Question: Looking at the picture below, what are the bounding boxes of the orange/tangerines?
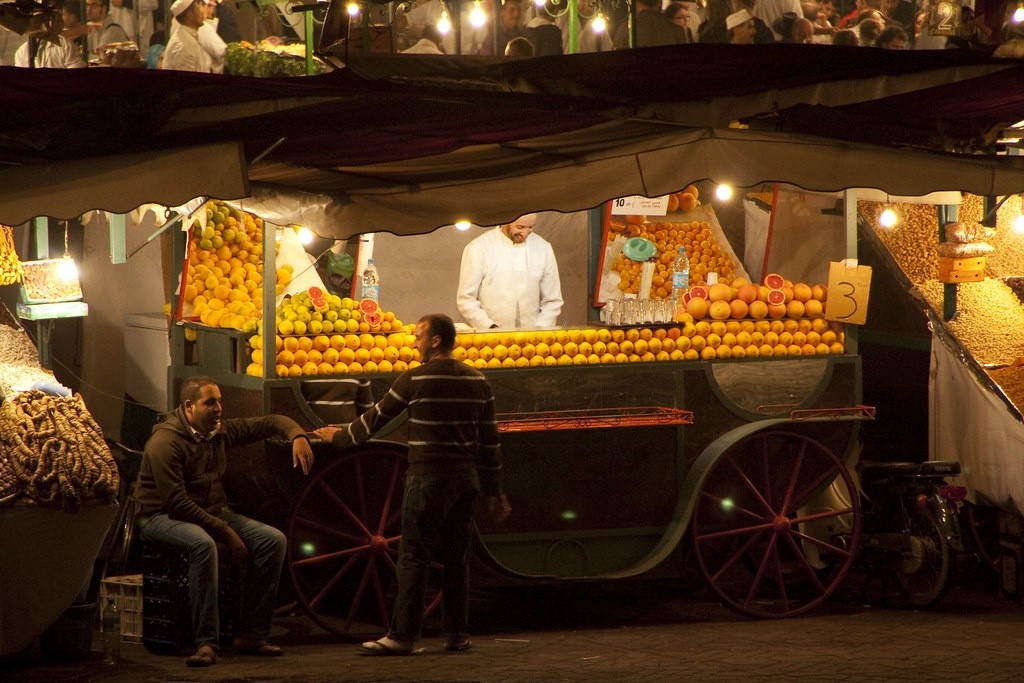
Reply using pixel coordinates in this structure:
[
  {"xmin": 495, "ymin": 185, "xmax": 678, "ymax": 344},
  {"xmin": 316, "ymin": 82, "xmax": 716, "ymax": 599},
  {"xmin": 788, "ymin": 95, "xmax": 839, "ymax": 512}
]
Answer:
[
  {"xmin": 608, "ymin": 184, "xmax": 738, "ymax": 298},
  {"xmin": 246, "ymin": 334, "xmax": 423, "ymax": 378},
  {"xmin": 448, "ymin": 319, "xmax": 846, "ymax": 369},
  {"xmin": 675, "ymin": 274, "xmax": 829, "ymax": 323},
  {"xmin": 182, "ymin": 201, "xmax": 416, "ymax": 333}
]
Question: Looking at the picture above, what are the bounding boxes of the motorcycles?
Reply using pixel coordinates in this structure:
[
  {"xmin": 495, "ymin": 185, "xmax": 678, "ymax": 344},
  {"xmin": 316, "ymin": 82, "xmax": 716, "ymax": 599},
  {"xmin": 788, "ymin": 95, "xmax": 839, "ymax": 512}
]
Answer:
[{"xmin": 737, "ymin": 461, "xmax": 967, "ymax": 613}]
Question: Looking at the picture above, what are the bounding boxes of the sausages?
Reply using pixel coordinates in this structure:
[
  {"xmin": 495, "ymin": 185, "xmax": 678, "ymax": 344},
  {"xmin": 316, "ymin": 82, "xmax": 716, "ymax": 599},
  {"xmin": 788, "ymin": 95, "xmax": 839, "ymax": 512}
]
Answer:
[{"xmin": 0, "ymin": 389, "xmax": 120, "ymax": 520}]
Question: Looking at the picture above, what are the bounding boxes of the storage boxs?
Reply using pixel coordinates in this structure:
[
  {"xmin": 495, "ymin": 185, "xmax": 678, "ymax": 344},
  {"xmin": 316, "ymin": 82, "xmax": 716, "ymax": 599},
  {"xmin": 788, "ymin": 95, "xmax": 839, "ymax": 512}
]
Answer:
[
  {"xmin": 97, "ymin": 571, "xmax": 142, "ymax": 646},
  {"xmin": 14, "ymin": 258, "xmax": 88, "ymax": 319},
  {"xmin": 939, "ymin": 257, "xmax": 986, "ymax": 284},
  {"xmin": 143, "ymin": 540, "xmax": 238, "ymax": 654}
]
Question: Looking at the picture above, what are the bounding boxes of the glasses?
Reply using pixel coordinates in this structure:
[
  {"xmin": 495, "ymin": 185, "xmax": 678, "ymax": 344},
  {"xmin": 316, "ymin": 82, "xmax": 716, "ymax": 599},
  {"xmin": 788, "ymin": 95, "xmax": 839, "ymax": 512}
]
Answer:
[{"xmin": 672, "ymin": 15, "xmax": 690, "ymax": 20}]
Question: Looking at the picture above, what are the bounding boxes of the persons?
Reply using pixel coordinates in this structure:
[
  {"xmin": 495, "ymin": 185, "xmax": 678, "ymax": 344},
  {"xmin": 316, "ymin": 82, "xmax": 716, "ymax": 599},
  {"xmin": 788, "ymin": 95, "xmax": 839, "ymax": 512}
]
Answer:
[
  {"xmin": 0, "ymin": 0, "xmax": 154, "ymax": 67},
  {"xmin": 395, "ymin": 0, "xmax": 774, "ymax": 56},
  {"xmin": 135, "ymin": 376, "xmax": 315, "ymax": 663},
  {"xmin": 753, "ymin": 0, "xmax": 1024, "ymax": 51},
  {"xmin": 457, "ymin": 212, "xmax": 564, "ymax": 329},
  {"xmin": 162, "ymin": 0, "xmax": 236, "ymax": 74},
  {"xmin": 314, "ymin": 317, "xmax": 511, "ymax": 654}
]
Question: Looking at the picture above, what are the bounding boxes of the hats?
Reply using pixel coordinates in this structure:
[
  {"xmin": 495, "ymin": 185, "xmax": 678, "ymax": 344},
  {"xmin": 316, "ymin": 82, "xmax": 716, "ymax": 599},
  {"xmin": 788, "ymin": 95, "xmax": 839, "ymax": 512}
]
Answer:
[
  {"xmin": 726, "ymin": 8, "xmax": 751, "ymax": 31},
  {"xmin": 170, "ymin": 0, "xmax": 194, "ymax": 16}
]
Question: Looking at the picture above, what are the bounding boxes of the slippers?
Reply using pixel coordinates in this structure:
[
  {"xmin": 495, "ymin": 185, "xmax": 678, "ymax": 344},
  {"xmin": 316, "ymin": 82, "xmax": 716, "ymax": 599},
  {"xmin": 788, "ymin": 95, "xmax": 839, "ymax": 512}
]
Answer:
[
  {"xmin": 233, "ymin": 640, "xmax": 284, "ymax": 656},
  {"xmin": 357, "ymin": 640, "xmax": 411, "ymax": 655},
  {"xmin": 185, "ymin": 650, "xmax": 217, "ymax": 667},
  {"xmin": 445, "ymin": 639, "xmax": 472, "ymax": 651}
]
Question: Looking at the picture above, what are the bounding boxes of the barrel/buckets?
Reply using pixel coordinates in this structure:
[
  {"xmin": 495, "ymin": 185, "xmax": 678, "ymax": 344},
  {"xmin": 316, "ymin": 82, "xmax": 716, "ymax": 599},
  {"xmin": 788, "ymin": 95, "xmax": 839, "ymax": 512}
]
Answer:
[{"xmin": 41, "ymin": 600, "xmax": 98, "ymax": 666}]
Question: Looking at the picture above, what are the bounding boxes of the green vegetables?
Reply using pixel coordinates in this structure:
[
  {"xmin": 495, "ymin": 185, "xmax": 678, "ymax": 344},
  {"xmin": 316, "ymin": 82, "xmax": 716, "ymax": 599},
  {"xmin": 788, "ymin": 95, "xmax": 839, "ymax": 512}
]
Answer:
[{"xmin": 224, "ymin": 42, "xmax": 325, "ymax": 76}]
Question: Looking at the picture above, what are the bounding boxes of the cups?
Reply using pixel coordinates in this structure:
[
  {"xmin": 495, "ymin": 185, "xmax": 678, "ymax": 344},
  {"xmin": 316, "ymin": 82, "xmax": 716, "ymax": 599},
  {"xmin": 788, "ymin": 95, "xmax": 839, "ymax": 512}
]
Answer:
[{"xmin": 606, "ymin": 298, "xmax": 678, "ymax": 325}]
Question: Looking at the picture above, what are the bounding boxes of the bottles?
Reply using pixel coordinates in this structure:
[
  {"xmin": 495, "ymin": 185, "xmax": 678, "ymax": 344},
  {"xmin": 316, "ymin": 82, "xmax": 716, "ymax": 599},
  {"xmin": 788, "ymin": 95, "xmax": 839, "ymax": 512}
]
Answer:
[
  {"xmin": 362, "ymin": 259, "xmax": 379, "ymax": 305},
  {"xmin": 102, "ymin": 597, "xmax": 121, "ymax": 665},
  {"xmin": 672, "ymin": 246, "xmax": 690, "ymax": 298},
  {"xmin": 934, "ymin": 490, "xmax": 961, "ymax": 551}
]
